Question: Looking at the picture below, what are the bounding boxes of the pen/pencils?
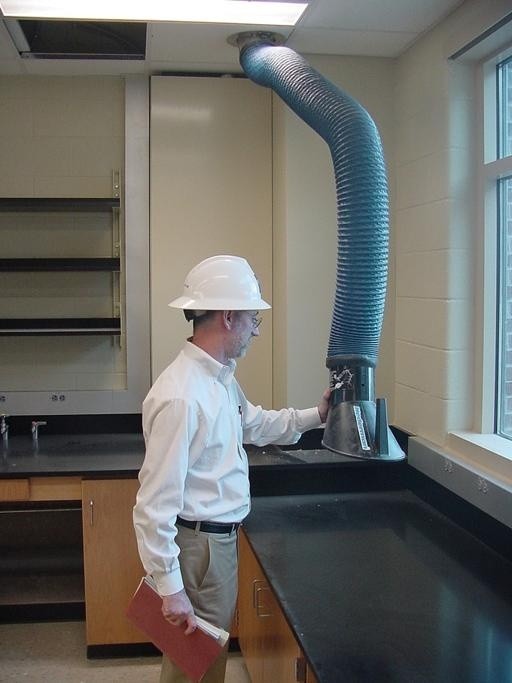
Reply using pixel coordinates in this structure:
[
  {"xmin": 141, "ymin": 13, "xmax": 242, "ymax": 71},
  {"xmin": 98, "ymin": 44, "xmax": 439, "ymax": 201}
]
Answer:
[{"xmin": 238, "ymin": 405, "xmax": 243, "ymax": 428}]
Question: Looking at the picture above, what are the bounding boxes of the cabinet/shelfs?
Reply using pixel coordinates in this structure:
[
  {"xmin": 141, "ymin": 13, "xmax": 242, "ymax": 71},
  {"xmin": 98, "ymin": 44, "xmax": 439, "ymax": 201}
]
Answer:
[
  {"xmin": 81, "ymin": 480, "xmax": 155, "ymax": 643},
  {"xmin": 0, "ymin": 74, "xmax": 125, "ymax": 335},
  {"xmin": 233, "ymin": 526, "xmax": 298, "ymax": 683}
]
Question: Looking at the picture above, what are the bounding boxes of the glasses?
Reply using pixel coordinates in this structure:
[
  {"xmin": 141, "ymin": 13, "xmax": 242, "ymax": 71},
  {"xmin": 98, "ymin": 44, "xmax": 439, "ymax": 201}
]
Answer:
[{"xmin": 242, "ymin": 311, "xmax": 263, "ymax": 328}]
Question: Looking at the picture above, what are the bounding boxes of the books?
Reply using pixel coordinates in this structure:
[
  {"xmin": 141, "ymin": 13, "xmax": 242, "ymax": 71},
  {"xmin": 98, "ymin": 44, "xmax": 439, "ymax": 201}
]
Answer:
[{"xmin": 122, "ymin": 572, "xmax": 230, "ymax": 683}]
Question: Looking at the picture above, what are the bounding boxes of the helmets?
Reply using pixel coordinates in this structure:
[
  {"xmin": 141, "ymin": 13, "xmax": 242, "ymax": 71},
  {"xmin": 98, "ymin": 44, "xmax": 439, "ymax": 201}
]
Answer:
[{"xmin": 167, "ymin": 253, "xmax": 274, "ymax": 314}]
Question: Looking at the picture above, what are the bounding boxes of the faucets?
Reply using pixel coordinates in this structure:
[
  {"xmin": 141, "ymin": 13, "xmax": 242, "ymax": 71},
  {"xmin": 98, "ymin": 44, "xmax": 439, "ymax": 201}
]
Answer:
[{"xmin": 0, "ymin": 415, "xmax": 9, "ymax": 441}]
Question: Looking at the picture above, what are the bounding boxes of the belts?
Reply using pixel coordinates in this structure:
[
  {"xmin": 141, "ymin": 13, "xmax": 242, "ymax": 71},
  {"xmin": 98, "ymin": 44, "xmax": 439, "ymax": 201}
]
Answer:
[{"xmin": 175, "ymin": 513, "xmax": 241, "ymax": 535}]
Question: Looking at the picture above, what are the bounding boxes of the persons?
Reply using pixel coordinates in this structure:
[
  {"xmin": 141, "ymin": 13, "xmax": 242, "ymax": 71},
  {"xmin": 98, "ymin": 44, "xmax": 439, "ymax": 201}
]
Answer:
[{"xmin": 131, "ymin": 252, "xmax": 334, "ymax": 683}]
